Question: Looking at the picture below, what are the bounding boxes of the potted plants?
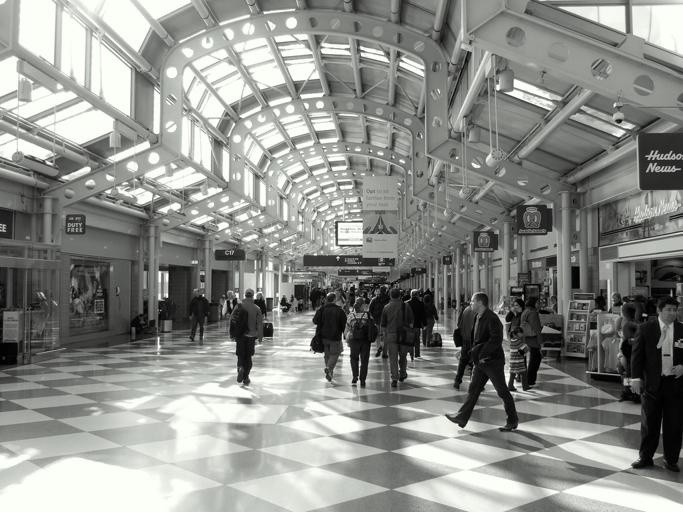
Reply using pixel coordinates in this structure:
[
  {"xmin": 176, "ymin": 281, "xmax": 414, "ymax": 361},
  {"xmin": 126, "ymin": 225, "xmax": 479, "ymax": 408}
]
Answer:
[{"xmin": 160, "ymin": 297, "xmax": 180, "ymax": 332}]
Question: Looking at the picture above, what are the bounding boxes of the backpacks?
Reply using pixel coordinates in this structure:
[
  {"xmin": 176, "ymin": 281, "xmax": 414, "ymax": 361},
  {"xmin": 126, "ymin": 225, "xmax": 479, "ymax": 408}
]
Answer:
[{"xmin": 353, "ymin": 312, "xmax": 366, "ymax": 340}]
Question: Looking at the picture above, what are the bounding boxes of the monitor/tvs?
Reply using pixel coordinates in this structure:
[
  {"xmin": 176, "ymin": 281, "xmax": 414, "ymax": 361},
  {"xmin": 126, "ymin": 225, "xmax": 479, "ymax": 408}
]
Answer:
[{"xmin": 335, "ymin": 220, "xmax": 364, "ymax": 246}]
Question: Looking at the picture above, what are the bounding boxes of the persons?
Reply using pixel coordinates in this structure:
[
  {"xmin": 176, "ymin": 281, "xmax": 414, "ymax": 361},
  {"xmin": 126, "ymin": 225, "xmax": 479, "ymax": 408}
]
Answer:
[
  {"xmin": 309, "ymin": 283, "xmax": 439, "ymax": 387},
  {"xmin": 229, "ymin": 288, "xmax": 264, "ymax": 385},
  {"xmin": 220, "ymin": 290, "xmax": 241, "ymax": 339},
  {"xmin": 590, "ymin": 296, "xmax": 606, "ymax": 329},
  {"xmin": 609, "ymin": 293, "xmax": 623, "ymax": 313},
  {"xmin": 254, "ymin": 292, "xmax": 267, "ymax": 320},
  {"xmin": 550, "ymin": 296, "xmax": 558, "ymax": 314},
  {"xmin": 444, "ymin": 292, "xmax": 519, "ymax": 431},
  {"xmin": 281, "ymin": 294, "xmax": 304, "ymax": 312},
  {"xmin": 632, "ymin": 296, "xmax": 683, "ymax": 473},
  {"xmin": 189, "ymin": 288, "xmax": 210, "ymax": 341},
  {"xmin": 506, "ymin": 296, "xmax": 543, "ymax": 390}
]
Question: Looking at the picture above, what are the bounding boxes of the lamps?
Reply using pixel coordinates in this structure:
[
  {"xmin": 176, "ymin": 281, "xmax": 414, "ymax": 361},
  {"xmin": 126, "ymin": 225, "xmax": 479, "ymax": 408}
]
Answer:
[
  {"xmin": 112, "ymin": 187, "xmax": 137, "ymax": 203},
  {"xmin": 17, "ymin": 78, "xmax": 31, "ymax": 101},
  {"xmin": 109, "ymin": 132, "xmax": 122, "ymax": 149},
  {"xmin": 14, "ymin": 150, "xmax": 60, "ymax": 178},
  {"xmin": 164, "ymin": 162, "xmax": 259, "ymax": 245},
  {"xmin": 399, "ymin": 65, "xmax": 515, "ymax": 268}
]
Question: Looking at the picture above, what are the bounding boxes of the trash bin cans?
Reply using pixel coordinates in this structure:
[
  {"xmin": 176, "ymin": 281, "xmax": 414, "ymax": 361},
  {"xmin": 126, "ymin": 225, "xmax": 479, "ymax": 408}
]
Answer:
[{"xmin": 208, "ymin": 303, "xmax": 221, "ymax": 321}]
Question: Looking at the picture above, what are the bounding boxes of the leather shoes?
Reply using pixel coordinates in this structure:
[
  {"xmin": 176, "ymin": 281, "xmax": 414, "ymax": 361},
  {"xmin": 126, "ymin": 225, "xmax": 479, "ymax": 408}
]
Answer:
[
  {"xmin": 445, "ymin": 414, "xmax": 466, "ymax": 428},
  {"xmin": 498, "ymin": 421, "xmax": 517, "ymax": 431},
  {"xmin": 663, "ymin": 458, "xmax": 680, "ymax": 473},
  {"xmin": 631, "ymin": 458, "xmax": 653, "ymax": 467}
]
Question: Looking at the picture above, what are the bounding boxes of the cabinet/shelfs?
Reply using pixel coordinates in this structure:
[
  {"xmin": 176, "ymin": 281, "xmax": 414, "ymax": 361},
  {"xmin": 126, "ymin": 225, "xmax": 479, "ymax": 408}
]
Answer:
[{"xmin": 564, "ymin": 301, "xmax": 595, "ymax": 358}]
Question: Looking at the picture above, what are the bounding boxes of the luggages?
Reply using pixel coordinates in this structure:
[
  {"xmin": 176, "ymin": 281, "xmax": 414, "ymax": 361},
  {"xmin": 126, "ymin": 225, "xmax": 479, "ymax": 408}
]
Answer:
[
  {"xmin": 263, "ymin": 319, "xmax": 273, "ymax": 337},
  {"xmin": 430, "ymin": 319, "xmax": 442, "ymax": 347}
]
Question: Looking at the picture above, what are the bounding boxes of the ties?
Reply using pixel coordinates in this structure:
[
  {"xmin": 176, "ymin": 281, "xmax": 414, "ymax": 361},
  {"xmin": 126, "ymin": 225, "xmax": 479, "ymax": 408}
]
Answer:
[{"xmin": 657, "ymin": 327, "xmax": 672, "ymax": 377}]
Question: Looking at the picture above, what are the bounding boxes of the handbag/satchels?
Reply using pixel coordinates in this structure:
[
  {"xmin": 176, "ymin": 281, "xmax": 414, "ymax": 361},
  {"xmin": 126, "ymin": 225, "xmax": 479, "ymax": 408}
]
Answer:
[
  {"xmin": 453, "ymin": 328, "xmax": 462, "ymax": 347},
  {"xmin": 402, "ymin": 329, "xmax": 416, "ymax": 346},
  {"xmin": 367, "ymin": 319, "xmax": 378, "ymax": 342},
  {"xmin": 311, "ymin": 325, "xmax": 324, "ymax": 352},
  {"xmin": 231, "ymin": 307, "xmax": 248, "ymax": 337}
]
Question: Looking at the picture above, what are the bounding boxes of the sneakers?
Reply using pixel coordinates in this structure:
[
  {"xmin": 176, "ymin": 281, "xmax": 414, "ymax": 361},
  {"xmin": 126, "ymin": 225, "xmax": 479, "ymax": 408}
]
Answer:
[
  {"xmin": 509, "ymin": 386, "xmax": 533, "ymax": 391},
  {"xmin": 352, "ymin": 376, "xmax": 358, "ymax": 383},
  {"xmin": 376, "ymin": 348, "xmax": 382, "ymax": 357},
  {"xmin": 383, "ymin": 354, "xmax": 388, "ymax": 358},
  {"xmin": 325, "ymin": 367, "xmax": 331, "ymax": 382},
  {"xmin": 400, "ymin": 373, "xmax": 407, "ymax": 382},
  {"xmin": 237, "ymin": 366, "xmax": 250, "ymax": 383},
  {"xmin": 190, "ymin": 335, "xmax": 193, "ymax": 340},
  {"xmin": 453, "ymin": 383, "xmax": 460, "ymax": 390},
  {"xmin": 391, "ymin": 380, "xmax": 397, "ymax": 386},
  {"xmin": 361, "ymin": 378, "xmax": 365, "ymax": 385}
]
